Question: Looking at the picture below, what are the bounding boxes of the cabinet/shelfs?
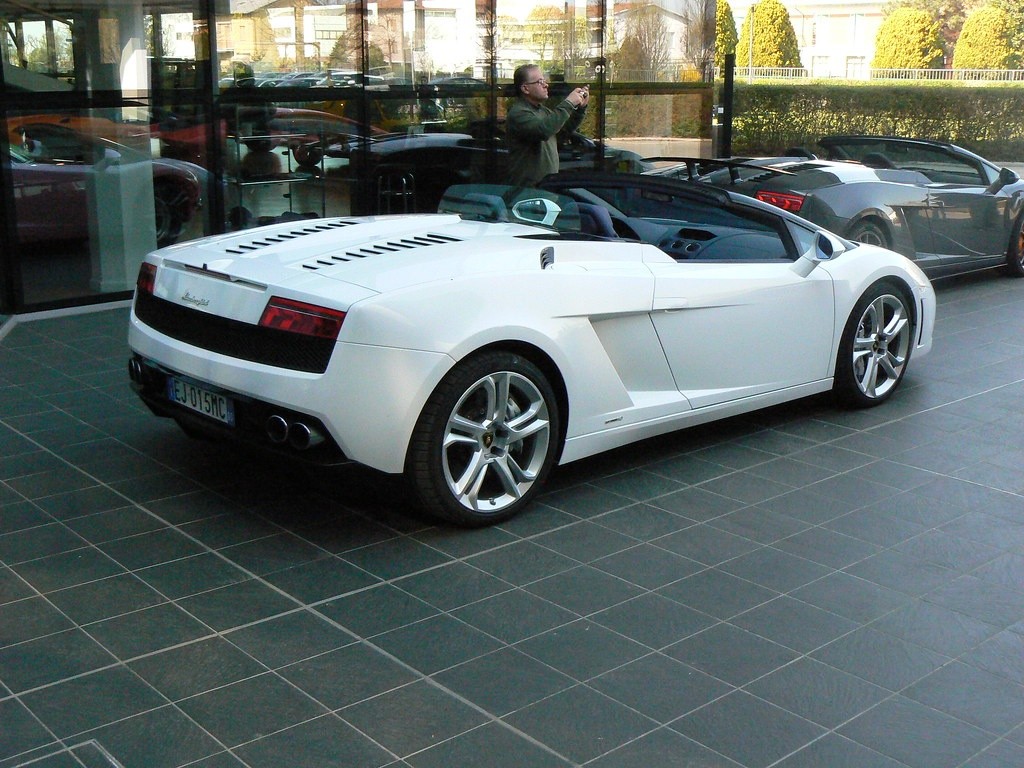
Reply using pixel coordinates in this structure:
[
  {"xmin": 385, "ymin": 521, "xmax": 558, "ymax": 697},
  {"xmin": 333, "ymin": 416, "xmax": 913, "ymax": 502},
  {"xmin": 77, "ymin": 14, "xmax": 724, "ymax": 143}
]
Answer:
[{"xmin": 225, "ymin": 38, "xmax": 326, "ymax": 223}]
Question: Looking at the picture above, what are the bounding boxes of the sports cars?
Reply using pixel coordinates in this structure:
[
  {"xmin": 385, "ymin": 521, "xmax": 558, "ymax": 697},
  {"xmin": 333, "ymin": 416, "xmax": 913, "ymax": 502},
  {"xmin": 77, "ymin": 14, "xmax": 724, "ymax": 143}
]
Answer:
[
  {"xmin": 1, "ymin": 71, "xmax": 656, "ymax": 251},
  {"xmin": 126, "ymin": 167, "xmax": 938, "ymax": 529},
  {"xmin": 638, "ymin": 136, "xmax": 1024, "ymax": 282}
]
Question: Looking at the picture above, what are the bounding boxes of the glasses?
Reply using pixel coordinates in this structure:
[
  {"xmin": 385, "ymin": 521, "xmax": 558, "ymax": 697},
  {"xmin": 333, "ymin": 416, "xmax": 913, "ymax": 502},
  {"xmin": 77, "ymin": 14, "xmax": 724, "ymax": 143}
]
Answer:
[{"xmin": 525, "ymin": 78, "xmax": 547, "ymax": 85}]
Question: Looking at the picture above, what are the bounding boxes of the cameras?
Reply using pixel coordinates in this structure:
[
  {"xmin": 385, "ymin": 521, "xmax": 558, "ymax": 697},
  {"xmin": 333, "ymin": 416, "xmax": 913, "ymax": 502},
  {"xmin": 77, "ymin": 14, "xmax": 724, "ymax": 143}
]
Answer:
[{"xmin": 580, "ymin": 91, "xmax": 588, "ymax": 99}]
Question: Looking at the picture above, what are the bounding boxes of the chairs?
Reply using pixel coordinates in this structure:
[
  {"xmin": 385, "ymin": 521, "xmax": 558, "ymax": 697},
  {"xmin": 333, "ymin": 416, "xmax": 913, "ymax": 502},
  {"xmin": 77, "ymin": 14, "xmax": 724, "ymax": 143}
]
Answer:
[
  {"xmin": 462, "ymin": 194, "xmax": 508, "ymax": 222},
  {"xmin": 553, "ymin": 202, "xmax": 620, "ymax": 238},
  {"xmin": 785, "ymin": 146, "xmax": 817, "ymax": 160},
  {"xmin": 861, "ymin": 153, "xmax": 897, "ymax": 170}
]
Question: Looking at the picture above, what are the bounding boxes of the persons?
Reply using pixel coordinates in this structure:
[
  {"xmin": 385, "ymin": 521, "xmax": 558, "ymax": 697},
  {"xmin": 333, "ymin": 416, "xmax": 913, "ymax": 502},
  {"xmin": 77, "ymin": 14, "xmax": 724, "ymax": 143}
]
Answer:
[
  {"xmin": 218, "ymin": 62, "xmax": 277, "ymax": 154},
  {"xmin": 417, "ymin": 76, "xmax": 440, "ymax": 120},
  {"xmin": 505, "ymin": 63, "xmax": 589, "ymax": 187}
]
